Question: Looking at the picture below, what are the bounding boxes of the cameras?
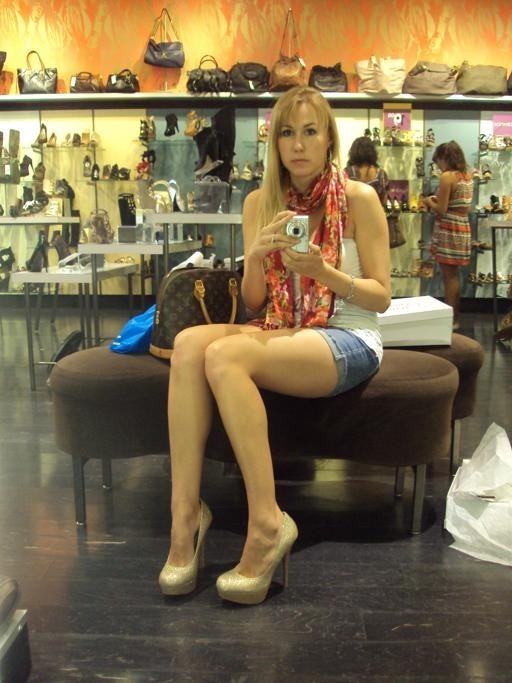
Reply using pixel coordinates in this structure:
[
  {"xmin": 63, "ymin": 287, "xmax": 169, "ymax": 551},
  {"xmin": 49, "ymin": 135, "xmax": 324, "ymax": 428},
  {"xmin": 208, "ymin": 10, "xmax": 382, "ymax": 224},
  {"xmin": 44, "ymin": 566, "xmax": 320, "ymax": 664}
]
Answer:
[{"xmin": 286, "ymin": 214, "xmax": 309, "ymax": 254}]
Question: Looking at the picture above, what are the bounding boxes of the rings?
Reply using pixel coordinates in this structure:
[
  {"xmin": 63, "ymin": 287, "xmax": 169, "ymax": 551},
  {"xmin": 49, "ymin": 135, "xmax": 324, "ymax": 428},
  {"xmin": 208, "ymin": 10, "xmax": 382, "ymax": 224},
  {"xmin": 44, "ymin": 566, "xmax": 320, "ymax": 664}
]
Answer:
[{"xmin": 271, "ymin": 232, "xmax": 274, "ymax": 241}]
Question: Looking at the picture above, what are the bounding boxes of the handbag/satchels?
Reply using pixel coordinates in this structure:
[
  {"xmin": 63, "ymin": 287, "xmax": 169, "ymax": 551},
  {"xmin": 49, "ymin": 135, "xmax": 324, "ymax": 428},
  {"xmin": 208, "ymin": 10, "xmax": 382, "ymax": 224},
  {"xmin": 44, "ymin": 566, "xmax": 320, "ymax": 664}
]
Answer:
[
  {"xmin": 226, "ymin": 62, "xmax": 269, "ymax": 93},
  {"xmin": 149, "ymin": 267, "xmax": 245, "ymax": 359},
  {"xmin": 268, "ymin": 8, "xmax": 306, "ymax": 93},
  {"xmin": 70, "ymin": 69, "xmax": 139, "ymax": 93},
  {"xmin": 144, "ymin": 8, "xmax": 184, "ymax": 67},
  {"xmin": 456, "ymin": 61, "xmax": 508, "ymax": 95},
  {"xmin": 187, "ymin": 55, "xmax": 228, "ymax": 93},
  {"xmin": 401, "ymin": 61, "xmax": 459, "ymax": 95},
  {"xmin": 386, "ymin": 210, "xmax": 405, "ymax": 248},
  {"xmin": 17, "ymin": 51, "xmax": 56, "ymax": 94},
  {"xmin": 357, "ymin": 55, "xmax": 406, "ymax": 94},
  {"xmin": 309, "ymin": 63, "xmax": 348, "ymax": 92}
]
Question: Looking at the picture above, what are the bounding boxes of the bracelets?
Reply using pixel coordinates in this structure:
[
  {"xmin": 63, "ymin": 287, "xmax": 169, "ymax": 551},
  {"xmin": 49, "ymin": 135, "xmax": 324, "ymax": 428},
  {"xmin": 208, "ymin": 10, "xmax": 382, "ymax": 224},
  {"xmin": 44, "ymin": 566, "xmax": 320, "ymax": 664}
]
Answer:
[{"xmin": 337, "ymin": 275, "xmax": 358, "ymax": 303}]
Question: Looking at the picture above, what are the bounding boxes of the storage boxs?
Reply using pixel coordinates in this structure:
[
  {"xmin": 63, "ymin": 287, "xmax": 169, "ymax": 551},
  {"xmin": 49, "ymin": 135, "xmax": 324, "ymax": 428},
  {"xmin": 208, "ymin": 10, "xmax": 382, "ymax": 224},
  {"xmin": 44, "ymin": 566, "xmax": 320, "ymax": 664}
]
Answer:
[{"xmin": 377, "ymin": 294, "xmax": 455, "ymax": 348}]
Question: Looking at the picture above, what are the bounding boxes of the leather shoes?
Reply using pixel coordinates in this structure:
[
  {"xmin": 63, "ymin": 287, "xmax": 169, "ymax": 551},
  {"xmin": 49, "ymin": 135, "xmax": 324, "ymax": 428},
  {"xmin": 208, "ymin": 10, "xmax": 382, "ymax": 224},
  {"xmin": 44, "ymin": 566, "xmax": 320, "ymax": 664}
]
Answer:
[
  {"xmin": 83, "ymin": 155, "xmax": 130, "ymax": 181},
  {"xmin": 38, "ymin": 124, "xmax": 90, "ymax": 147},
  {"xmin": 231, "ymin": 160, "xmax": 263, "ymax": 181},
  {"xmin": 8, "ymin": 183, "xmax": 48, "ymax": 216}
]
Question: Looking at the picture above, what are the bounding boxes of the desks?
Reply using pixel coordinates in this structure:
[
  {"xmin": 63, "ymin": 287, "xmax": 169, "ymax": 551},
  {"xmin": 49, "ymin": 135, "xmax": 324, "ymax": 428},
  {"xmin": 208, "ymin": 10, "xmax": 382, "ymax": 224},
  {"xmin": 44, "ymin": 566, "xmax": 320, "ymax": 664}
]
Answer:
[
  {"xmin": 490, "ymin": 222, "xmax": 512, "ymax": 332},
  {"xmin": 10, "ymin": 214, "xmax": 79, "ymax": 331},
  {"xmin": 78, "ymin": 240, "xmax": 203, "ymax": 352},
  {"xmin": 11, "ymin": 261, "xmax": 139, "ymax": 392},
  {"xmin": 145, "ymin": 211, "xmax": 247, "ymax": 276}
]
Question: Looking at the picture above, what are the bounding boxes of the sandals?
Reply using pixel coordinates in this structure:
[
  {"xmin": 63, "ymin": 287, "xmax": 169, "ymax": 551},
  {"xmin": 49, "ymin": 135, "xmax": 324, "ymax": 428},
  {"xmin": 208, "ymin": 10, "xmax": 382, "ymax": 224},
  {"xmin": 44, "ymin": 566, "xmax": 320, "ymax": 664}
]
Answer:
[
  {"xmin": 472, "ymin": 194, "xmax": 512, "ymax": 218},
  {"xmin": 386, "ymin": 195, "xmax": 442, "ymax": 213},
  {"xmin": 391, "ymin": 263, "xmax": 512, "ymax": 284},
  {"xmin": 470, "ymin": 240, "xmax": 492, "ymax": 254},
  {"xmin": 479, "ymin": 133, "xmax": 512, "ymax": 151},
  {"xmin": 416, "ymin": 157, "xmax": 492, "ymax": 178},
  {"xmin": 365, "ymin": 126, "xmax": 434, "ymax": 146}
]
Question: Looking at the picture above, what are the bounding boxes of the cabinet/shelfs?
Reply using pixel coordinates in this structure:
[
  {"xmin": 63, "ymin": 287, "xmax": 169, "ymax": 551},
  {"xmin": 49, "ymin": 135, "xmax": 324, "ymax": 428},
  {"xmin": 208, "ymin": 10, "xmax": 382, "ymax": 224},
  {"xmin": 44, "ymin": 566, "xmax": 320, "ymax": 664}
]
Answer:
[{"xmin": 0, "ymin": 87, "xmax": 512, "ymax": 286}]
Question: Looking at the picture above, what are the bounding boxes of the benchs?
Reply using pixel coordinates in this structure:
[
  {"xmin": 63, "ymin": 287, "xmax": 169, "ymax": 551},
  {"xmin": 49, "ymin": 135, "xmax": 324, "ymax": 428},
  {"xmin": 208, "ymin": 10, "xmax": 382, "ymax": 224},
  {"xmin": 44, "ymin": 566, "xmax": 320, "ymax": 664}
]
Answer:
[
  {"xmin": 46, "ymin": 350, "xmax": 460, "ymax": 536},
  {"xmin": 224, "ymin": 329, "xmax": 484, "ymax": 468}
]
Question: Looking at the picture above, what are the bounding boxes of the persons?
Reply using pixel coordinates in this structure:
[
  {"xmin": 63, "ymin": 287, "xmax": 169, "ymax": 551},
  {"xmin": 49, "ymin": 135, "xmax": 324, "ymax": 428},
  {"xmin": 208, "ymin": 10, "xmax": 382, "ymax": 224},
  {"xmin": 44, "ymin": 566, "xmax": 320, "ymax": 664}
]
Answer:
[
  {"xmin": 156, "ymin": 82, "xmax": 392, "ymax": 606},
  {"xmin": 342, "ymin": 133, "xmax": 390, "ymax": 216},
  {"xmin": 419, "ymin": 139, "xmax": 475, "ymax": 330}
]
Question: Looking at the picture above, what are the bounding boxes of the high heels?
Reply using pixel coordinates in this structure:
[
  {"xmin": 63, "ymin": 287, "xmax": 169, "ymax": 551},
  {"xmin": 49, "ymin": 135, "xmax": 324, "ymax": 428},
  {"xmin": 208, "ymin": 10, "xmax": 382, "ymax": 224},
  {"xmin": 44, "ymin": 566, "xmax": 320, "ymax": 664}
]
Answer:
[
  {"xmin": 20, "ymin": 155, "xmax": 45, "ymax": 180},
  {"xmin": 158, "ymin": 498, "xmax": 212, "ymax": 595},
  {"xmin": 26, "ymin": 235, "xmax": 74, "ymax": 272},
  {"xmin": 194, "ymin": 131, "xmax": 217, "ymax": 173},
  {"xmin": 150, "ymin": 180, "xmax": 185, "ymax": 213},
  {"xmin": 216, "ymin": 511, "xmax": 298, "ymax": 604},
  {"xmin": 140, "ymin": 111, "xmax": 201, "ymax": 141}
]
